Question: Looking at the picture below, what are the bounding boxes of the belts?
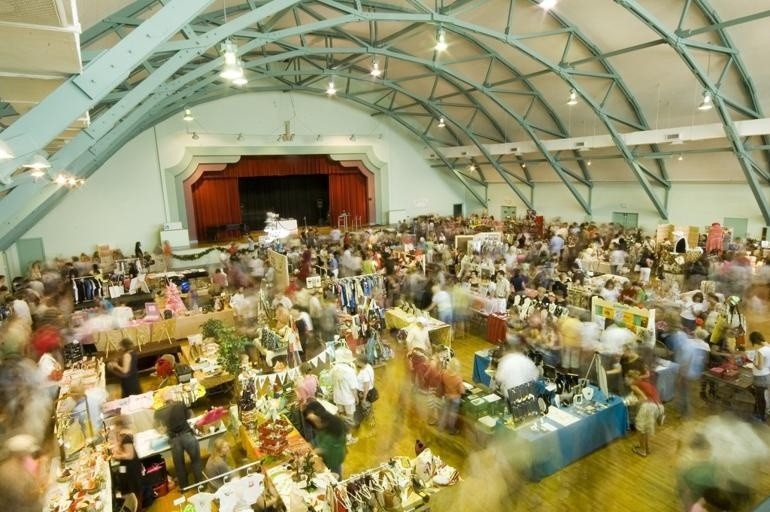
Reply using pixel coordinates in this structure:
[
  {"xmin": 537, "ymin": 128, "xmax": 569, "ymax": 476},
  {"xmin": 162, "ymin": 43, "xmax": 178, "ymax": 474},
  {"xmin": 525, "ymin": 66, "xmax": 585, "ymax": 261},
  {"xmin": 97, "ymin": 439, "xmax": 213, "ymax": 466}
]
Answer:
[{"xmin": 171, "ymin": 428, "xmax": 191, "ymax": 439}]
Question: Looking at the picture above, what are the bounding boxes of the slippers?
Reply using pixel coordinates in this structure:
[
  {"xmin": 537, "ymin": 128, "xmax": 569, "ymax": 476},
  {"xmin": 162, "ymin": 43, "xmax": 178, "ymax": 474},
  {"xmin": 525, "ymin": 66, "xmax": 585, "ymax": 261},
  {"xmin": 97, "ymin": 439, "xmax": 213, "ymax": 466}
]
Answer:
[{"xmin": 632, "ymin": 444, "xmax": 652, "ymax": 457}]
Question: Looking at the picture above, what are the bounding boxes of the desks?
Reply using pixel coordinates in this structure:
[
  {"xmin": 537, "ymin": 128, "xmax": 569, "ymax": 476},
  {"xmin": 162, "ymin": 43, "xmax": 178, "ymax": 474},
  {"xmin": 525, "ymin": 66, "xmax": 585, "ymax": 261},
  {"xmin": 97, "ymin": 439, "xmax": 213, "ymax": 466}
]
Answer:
[
  {"xmin": 460, "ymin": 286, "xmax": 681, "ymax": 478},
  {"xmin": 42, "ymin": 285, "xmax": 465, "ymax": 511},
  {"xmin": 700, "ymin": 353, "xmax": 753, "ymax": 405}
]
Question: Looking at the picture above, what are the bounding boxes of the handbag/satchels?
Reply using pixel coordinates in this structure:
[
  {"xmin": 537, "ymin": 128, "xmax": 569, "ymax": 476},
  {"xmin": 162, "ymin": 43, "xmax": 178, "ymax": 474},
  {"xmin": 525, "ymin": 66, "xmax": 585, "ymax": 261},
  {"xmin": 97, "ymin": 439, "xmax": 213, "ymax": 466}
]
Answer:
[
  {"xmin": 332, "ymin": 469, "xmax": 402, "ymax": 512},
  {"xmin": 367, "ymin": 389, "xmax": 379, "ymax": 402},
  {"xmin": 351, "ymin": 299, "xmax": 386, "ymax": 340},
  {"xmin": 657, "ymin": 414, "xmax": 666, "ymax": 426},
  {"xmin": 710, "ymin": 313, "xmax": 746, "ymax": 352}
]
